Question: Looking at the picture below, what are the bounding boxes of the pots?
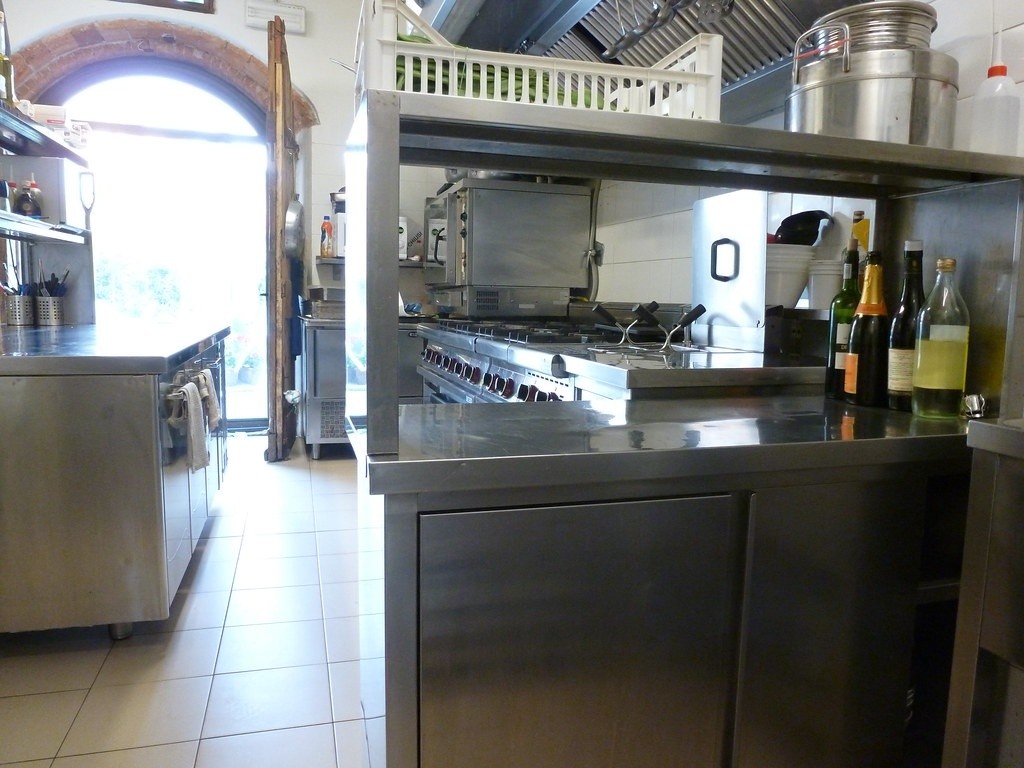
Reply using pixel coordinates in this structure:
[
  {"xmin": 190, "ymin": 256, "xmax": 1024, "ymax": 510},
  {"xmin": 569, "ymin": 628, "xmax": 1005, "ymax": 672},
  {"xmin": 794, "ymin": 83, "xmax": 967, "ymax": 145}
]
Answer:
[{"xmin": 783, "ymin": 21, "xmax": 959, "ymax": 150}]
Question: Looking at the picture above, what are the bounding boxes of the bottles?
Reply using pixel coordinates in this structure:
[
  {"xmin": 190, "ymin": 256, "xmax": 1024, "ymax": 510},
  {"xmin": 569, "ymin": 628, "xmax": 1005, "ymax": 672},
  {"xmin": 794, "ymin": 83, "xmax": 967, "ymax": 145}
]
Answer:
[
  {"xmin": 970, "ymin": 23, "xmax": 1020, "ymax": 157},
  {"xmin": 0, "ymin": 52, "xmax": 12, "ymax": 106},
  {"xmin": 320, "ymin": 215, "xmax": 334, "ymax": 257},
  {"xmin": 28, "ymin": 173, "xmax": 43, "ymax": 213},
  {"xmin": 887, "ymin": 240, "xmax": 934, "ymax": 410},
  {"xmin": 840, "ymin": 211, "xmax": 870, "ymax": 294},
  {"xmin": 843, "ymin": 251, "xmax": 892, "ymax": 407},
  {"xmin": 824, "ymin": 251, "xmax": 864, "ymax": 398},
  {"xmin": 911, "ymin": 258, "xmax": 970, "ymax": 419},
  {"xmin": 8, "ymin": 182, "xmax": 19, "ymax": 212},
  {"xmin": 17, "ymin": 188, "xmax": 35, "ymax": 216}
]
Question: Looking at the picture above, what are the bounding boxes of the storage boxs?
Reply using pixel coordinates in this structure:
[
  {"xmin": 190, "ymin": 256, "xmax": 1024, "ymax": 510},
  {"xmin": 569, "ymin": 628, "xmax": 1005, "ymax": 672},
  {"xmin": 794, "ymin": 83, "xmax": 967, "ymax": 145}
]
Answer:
[{"xmin": 353, "ymin": 1, "xmax": 724, "ymax": 124}]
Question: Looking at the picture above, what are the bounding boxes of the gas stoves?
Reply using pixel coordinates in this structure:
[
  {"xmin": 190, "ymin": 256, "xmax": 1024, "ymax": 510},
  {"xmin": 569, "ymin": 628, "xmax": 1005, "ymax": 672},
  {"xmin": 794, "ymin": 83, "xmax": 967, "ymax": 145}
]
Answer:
[{"xmin": 471, "ymin": 319, "xmax": 620, "ymax": 347}]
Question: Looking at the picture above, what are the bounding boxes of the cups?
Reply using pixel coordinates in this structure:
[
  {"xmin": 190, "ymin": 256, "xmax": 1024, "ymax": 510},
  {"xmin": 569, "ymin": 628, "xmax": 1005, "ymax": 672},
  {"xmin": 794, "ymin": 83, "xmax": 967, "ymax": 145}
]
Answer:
[{"xmin": 809, "ymin": 259, "xmax": 845, "ymax": 310}]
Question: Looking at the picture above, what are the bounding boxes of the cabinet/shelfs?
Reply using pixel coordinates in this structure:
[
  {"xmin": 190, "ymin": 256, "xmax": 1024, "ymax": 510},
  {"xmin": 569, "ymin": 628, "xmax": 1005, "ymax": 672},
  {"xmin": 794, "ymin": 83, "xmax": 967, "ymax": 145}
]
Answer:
[
  {"xmin": 417, "ymin": 472, "xmax": 971, "ymax": 768},
  {"xmin": 0, "ymin": 341, "xmax": 227, "ymax": 633},
  {"xmin": 688, "ymin": 190, "xmax": 768, "ymax": 354},
  {"xmin": 0, "ymin": 98, "xmax": 91, "ymax": 244}
]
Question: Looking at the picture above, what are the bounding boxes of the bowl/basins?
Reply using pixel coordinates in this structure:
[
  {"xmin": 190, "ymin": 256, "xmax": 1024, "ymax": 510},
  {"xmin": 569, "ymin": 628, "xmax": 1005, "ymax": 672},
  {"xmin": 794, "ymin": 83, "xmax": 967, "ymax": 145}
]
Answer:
[{"xmin": 766, "ymin": 243, "xmax": 822, "ymax": 309}]
